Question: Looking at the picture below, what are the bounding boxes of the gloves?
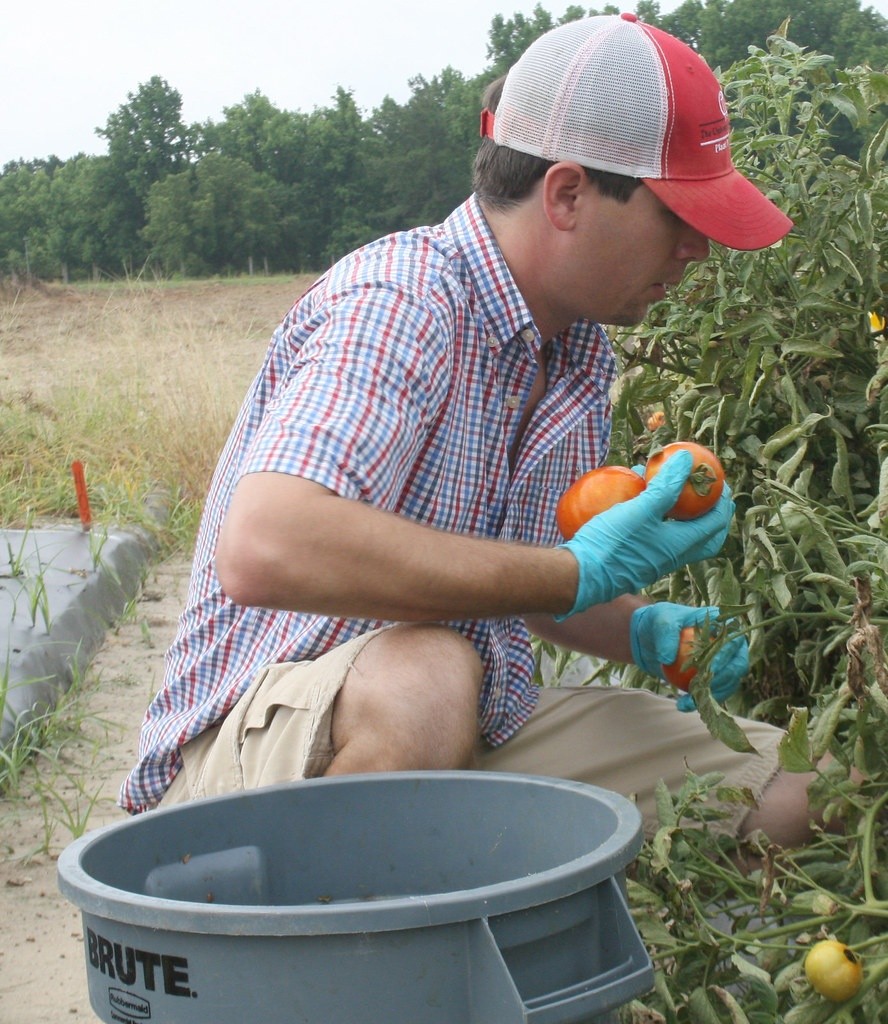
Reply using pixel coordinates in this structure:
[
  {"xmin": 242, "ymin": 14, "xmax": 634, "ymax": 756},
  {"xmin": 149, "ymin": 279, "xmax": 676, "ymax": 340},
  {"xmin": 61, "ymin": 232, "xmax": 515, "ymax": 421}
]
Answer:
[
  {"xmin": 552, "ymin": 449, "xmax": 736, "ymax": 624},
  {"xmin": 630, "ymin": 601, "xmax": 750, "ymax": 714}
]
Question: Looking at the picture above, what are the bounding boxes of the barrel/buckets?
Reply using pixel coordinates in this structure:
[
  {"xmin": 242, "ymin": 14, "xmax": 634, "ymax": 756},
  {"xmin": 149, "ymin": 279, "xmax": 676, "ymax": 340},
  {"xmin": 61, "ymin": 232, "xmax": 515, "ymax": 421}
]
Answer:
[{"xmin": 61, "ymin": 767, "xmax": 655, "ymax": 1024}]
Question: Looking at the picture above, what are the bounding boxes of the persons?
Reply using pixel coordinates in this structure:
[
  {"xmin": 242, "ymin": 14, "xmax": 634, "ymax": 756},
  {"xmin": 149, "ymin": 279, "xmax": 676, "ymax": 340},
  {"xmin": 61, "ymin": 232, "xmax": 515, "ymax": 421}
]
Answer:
[{"xmin": 114, "ymin": 13, "xmax": 861, "ymax": 882}]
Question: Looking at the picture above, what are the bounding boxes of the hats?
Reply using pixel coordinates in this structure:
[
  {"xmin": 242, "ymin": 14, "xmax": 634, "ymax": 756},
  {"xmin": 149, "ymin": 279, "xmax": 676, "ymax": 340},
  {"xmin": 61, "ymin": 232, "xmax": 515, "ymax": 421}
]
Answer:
[{"xmin": 478, "ymin": 12, "xmax": 795, "ymax": 251}]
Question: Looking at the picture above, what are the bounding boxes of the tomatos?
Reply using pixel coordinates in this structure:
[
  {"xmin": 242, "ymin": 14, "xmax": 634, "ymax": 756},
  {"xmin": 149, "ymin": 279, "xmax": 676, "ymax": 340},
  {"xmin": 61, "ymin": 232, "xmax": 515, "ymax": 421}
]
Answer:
[
  {"xmin": 557, "ymin": 466, "xmax": 646, "ymax": 540},
  {"xmin": 805, "ymin": 940, "xmax": 863, "ymax": 1000},
  {"xmin": 666, "ymin": 627, "xmax": 717, "ymax": 693},
  {"xmin": 645, "ymin": 442, "xmax": 724, "ymax": 521}
]
